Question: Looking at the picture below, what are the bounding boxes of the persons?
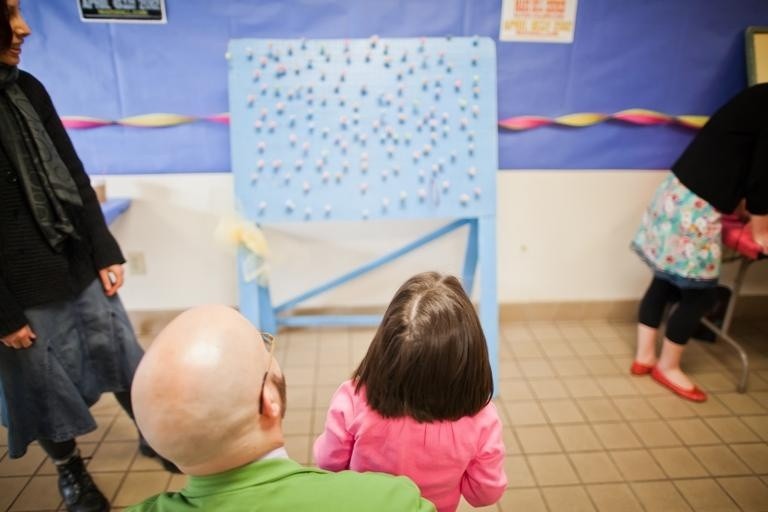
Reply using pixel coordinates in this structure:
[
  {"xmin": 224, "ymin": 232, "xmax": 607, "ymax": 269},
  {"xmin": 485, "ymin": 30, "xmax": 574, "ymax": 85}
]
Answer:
[
  {"xmin": 628, "ymin": 82, "xmax": 768, "ymax": 403},
  {"xmin": 312, "ymin": 269, "xmax": 508, "ymax": 512},
  {"xmin": 0, "ymin": 0, "xmax": 184, "ymax": 512},
  {"xmin": 121, "ymin": 302, "xmax": 438, "ymax": 512}
]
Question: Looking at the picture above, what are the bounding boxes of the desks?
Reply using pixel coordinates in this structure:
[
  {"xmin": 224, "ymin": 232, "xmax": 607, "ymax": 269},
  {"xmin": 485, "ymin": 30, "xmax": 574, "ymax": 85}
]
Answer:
[
  {"xmin": 662, "ymin": 213, "xmax": 768, "ymax": 396},
  {"xmin": 93, "ymin": 192, "xmax": 133, "ymax": 227}
]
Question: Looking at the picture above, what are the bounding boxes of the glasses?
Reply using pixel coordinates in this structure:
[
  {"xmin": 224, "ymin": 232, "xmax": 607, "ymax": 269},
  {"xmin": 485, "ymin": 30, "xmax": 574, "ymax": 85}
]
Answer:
[{"xmin": 260, "ymin": 330, "xmax": 277, "ymax": 418}]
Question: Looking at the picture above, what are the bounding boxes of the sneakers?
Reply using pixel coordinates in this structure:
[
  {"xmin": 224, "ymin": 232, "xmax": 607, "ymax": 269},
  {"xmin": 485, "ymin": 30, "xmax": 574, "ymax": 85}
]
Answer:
[
  {"xmin": 57, "ymin": 448, "xmax": 112, "ymax": 511},
  {"xmin": 652, "ymin": 366, "xmax": 707, "ymax": 403},
  {"xmin": 139, "ymin": 436, "xmax": 186, "ymax": 476},
  {"xmin": 631, "ymin": 360, "xmax": 654, "ymax": 375}
]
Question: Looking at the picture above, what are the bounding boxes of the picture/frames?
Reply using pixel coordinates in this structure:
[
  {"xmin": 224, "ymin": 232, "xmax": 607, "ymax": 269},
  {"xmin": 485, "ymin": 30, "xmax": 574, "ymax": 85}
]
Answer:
[{"xmin": 75, "ymin": 1, "xmax": 167, "ymax": 26}]
